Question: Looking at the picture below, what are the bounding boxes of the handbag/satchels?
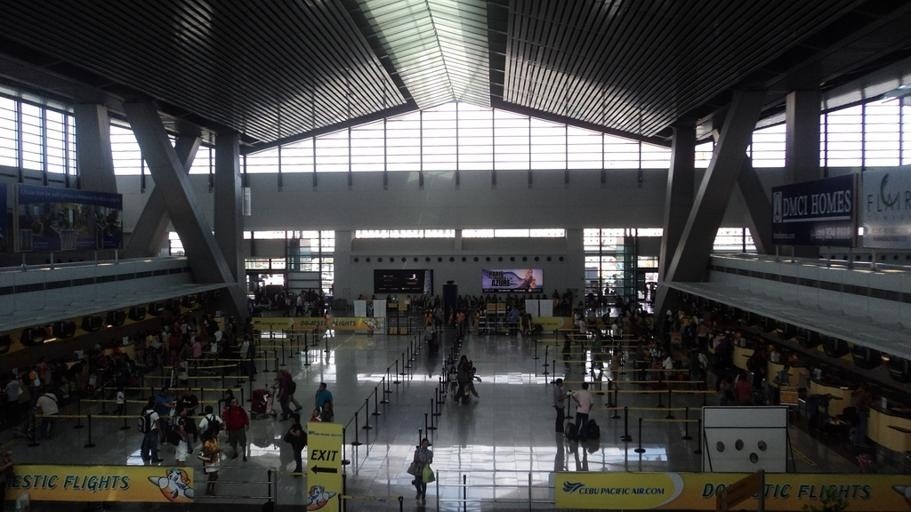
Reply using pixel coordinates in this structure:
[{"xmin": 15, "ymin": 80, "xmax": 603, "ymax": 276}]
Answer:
[
  {"xmin": 581, "ymin": 419, "xmax": 600, "ymax": 440},
  {"xmin": 408, "ymin": 463, "xmax": 420, "ymax": 476},
  {"xmin": 423, "ymin": 463, "xmax": 435, "ymax": 483},
  {"xmin": 565, "ymin": 423, "xmax": 577, "ymax": 439},
  {"xmin": 197, "ymin": 449, "xmax": 213, "ymax": 462},
  {"xmin": 284, "ymin": 430, "xmax": 307, "ymax": 445}
]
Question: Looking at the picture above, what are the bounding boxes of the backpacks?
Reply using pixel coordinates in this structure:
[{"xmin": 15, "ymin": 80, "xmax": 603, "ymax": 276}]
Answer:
[
  {"xmin": 137, "ymin": 411, "xmax": 155, "ymax": 433},
  {"xmin": 286, "ymin": 376, "xmax": 296, "ymax": 395},
  {"xmin": 204, "ymin": 414, "xmax": 220, "ymax": 436}
]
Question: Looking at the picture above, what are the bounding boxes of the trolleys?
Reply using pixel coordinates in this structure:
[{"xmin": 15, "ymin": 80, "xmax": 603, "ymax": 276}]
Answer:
[{"xmin": 247, "ymin": 384, "xmax": 279, "ymax": 421}]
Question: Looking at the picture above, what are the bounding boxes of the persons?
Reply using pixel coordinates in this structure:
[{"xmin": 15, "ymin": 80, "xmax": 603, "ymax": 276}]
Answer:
[
  {"xmin": 572, "ymin": 383, "xmax": 593, "ymax": 442},
  {"xmin": 141, "ymin": 387, "xmax": 200, "ymax": 467},
  {"xmin": 412, "ymin": 438, "xmax": 433, "ymax": 508},
  {"xmin": 528, "ymin": 279, "xmax": 537, "ymax": 288},
  {"xmin": 554, "ymin": 378, "xmax": 569, "ymax": 433},
  {"xmin": 277, "ymin": 371, "xmax": 302, "ymax": 421},
  {"xmin": 222, "ymin": 390, "xmax": 250, "ymax": 461},
  {"xmin": 809, "ymin": 393, "xmax": 833, "ymax": 436},
  {"xmin": 446, "ymin": 355, "xmax": 483, "ymax": 406},
  {"xmin": 0, "ymin": 314, "xmax": 254, "ymax": 438},
  {"xmin": 289, "ymin": 413, "xmax": 305, "ymax": 477},
  {"xmin": 310, "ymin": 383, "xmax": 332, "ymax": 422},
  {"xmin": 355, "ymin": 289, "xmax": 548, "ymax": 361},
  {"xmin": 552, "ymin": 293, "xmax": 672, "ymax": 382},
  {"xmin": 517, "ymin": 269, "xmax": 533, "ymax": 289},
  {"xmin": 853, "ymin": 383, "xmax": 872, "ymax": 451},
  {"xmin": 199, "ymin": 406, "xmax": 225, "ymax": 496},
  {"xmin": 677, "ymin": 309, "xmax": 887, "ymax": 420},
  {"xmin": 248, "ymin": 289, "xmax": 333, "ymax": 317}
]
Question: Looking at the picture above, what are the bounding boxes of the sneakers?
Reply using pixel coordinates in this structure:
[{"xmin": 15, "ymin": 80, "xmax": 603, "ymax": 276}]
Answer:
[
  {"xmin": 417, "ymin": 493, "xmax": 426, "ymax": 505},
  {"xmin": 205, "ymin": 489, "xmax": 216, "ymax": 496},
  {"xmin": 291, "ymin": 470, "xmax": 303, "ymax": 478},
  {"xmin": 555, "ymin": 428, "xmax": 587, "ymax": 442},
  {"xmin": 143, "ymin": 456, "xmax": 164, "ymax": 463},
  {"xmin": 451, "ymin": 395, "xmax": 480, "ymax": 405},
  {"xmin": 231, "ymin": 452, "xmax": 247, "ymax": 462},
  {"xmin": 279, "ymin": 407, "xmax": 302, "ymax": 422}
]
